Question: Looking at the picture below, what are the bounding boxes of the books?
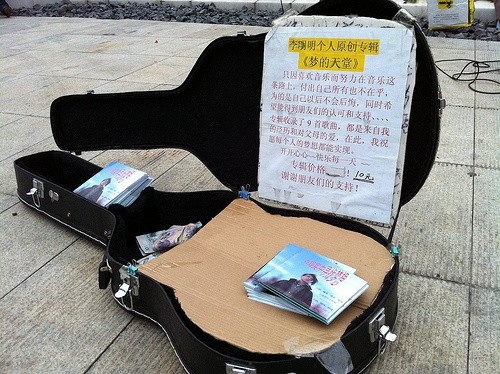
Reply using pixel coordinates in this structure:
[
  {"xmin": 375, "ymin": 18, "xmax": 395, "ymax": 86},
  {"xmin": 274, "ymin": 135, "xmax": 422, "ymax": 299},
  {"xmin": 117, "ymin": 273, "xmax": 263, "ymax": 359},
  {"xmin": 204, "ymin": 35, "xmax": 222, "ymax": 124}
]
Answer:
[
  {"xmin": 243, "ymin": 244, "xmax": 370, "ymax": 324},
  {"xmin": 73, "ymin": 160, "xmax": 157, "ymax": 213}
]
